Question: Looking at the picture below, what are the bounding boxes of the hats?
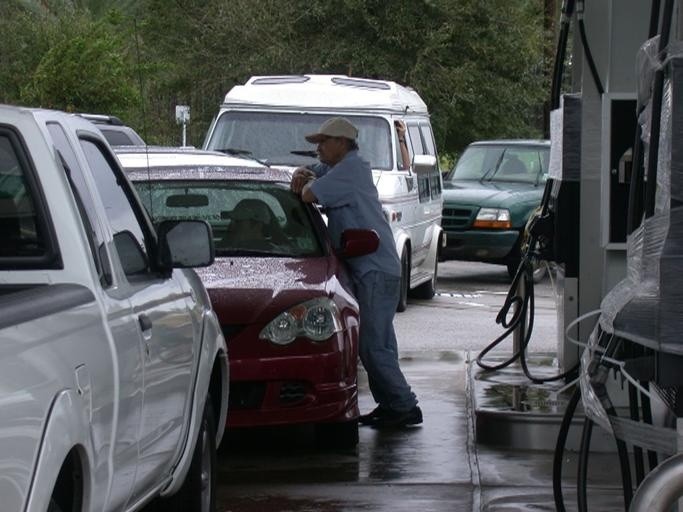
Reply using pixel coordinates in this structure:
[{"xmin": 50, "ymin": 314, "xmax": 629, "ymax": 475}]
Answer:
[
  {"xmin": 220, "ymin": 198, "xmax": 272, "ymax": 226},
  {"xmin": 305, "ymin": 117, "xmax": 358, "ymax": 143}
]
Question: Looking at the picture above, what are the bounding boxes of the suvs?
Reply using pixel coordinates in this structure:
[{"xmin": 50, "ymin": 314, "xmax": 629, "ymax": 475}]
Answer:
[
  {"xmin": 70, "ymin": 111, "xmax": 150, "ymax": 145},
  {"xmin": 439, "ymin": 140, "xmax": 548, "ymax": 283}
]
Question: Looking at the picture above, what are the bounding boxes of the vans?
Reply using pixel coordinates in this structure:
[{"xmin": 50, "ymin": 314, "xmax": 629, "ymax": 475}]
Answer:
[{"xmin": 203, "ymin": 72, "xmax": 445, "ymax": 308}]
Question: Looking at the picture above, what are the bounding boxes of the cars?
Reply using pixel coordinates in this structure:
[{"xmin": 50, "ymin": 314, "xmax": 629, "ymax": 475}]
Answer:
[{"xmin": 115, "ymin": 146, "xmax": 381, "ymax": 449}]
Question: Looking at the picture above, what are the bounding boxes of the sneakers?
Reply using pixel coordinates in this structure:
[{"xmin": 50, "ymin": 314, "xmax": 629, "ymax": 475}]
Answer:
[{"xmin": 358, "ymin": 405, "xmax": 423, "ymax": 426}]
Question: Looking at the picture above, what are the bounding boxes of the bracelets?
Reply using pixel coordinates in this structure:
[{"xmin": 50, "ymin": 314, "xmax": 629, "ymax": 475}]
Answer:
[{"xmin": 399, "ymin": 139, "xmax": 406, "ymax": 143}]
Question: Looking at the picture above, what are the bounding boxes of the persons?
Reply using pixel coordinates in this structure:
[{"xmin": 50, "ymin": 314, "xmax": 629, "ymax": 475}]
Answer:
[
  {"xmin": 220, "ymin": 198, "xmax": 281, "ymax": 252},
  {"xmin": 372, "ymin": 120, "xmax": 411, "ymax": 171},
  {"xmin": 291, "ymin": 117, "xmax": 423, "ymax": 430}
]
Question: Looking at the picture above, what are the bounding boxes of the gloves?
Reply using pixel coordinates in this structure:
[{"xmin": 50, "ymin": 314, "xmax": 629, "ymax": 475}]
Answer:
[{"xmin": 396, "ymin": 120, "xmax": 406, "ymax": 143}]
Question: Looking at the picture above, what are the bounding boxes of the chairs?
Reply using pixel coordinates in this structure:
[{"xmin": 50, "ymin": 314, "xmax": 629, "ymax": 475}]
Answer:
[
  {"xmin": 219, "ymin": 196, "xmax": 288, "ymax": 250},
  {"xmin": 496, "ymin": 155, "xmax": 530, "ymax": 176}
]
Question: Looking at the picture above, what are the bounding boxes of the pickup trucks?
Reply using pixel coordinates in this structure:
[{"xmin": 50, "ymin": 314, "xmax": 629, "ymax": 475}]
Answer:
[{"xmin": 0, "ymin": 105, "xmax": 232, "ymax": 512}]
